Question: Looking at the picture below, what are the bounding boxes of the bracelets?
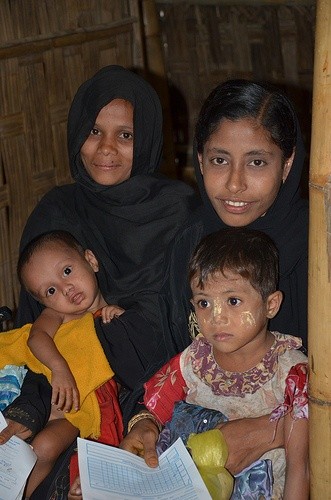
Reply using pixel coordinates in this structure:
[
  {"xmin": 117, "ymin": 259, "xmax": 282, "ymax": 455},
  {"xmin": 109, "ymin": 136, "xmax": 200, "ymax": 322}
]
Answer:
[{"xmin": 128, "ymin": 412, "xmax": 163, "ymax": 435}]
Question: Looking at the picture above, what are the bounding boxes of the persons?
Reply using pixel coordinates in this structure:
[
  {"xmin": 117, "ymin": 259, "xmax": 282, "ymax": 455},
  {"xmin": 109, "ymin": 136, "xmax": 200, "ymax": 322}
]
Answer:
[
  {"xmin": 0, "ymin": 65, "xmax": 196, "ymax": 500},
  {"xmin": 143, "ymin": 227, "xmax": 312, "ymax": 500},
  {"xmin": 118, "ymin": 76, "xmax": 310, "ymax": 468},
  {"xmin": 16, "ymin": 228, "xmax": 126, "ymax": 500}
]
